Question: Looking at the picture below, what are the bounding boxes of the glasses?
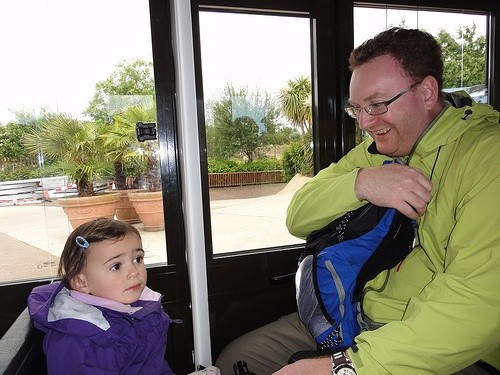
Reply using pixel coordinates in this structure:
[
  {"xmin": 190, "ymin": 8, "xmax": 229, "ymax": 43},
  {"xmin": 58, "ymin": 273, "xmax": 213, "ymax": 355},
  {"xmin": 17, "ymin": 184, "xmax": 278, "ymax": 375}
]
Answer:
[{"xmin": 344, "ymin": 80, "xmax": 422, "ymax": 119}]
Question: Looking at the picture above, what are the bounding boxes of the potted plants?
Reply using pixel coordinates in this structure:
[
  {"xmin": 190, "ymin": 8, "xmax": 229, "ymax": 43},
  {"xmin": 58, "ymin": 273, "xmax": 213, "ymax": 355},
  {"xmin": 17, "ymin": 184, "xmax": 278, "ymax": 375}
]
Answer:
[{"xmin": 22, "ymin": 58, "xmax": 164, "ymax": 231}]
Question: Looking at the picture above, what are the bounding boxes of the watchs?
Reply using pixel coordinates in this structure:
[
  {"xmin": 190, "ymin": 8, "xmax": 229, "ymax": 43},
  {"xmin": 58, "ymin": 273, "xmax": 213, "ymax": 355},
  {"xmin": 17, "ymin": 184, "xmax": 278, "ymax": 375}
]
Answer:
[{"xmin": 331, "ymin": 351, "xmax": 358, "ymax": 375}]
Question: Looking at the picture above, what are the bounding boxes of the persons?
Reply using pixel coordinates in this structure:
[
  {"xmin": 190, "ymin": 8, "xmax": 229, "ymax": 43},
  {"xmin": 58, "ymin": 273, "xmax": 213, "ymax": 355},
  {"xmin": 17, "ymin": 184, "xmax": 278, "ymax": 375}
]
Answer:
[
  {"xmin": 217, "ymin": 28, "xmax": 500, "ymax": 375},
  {"xmin": 27, "ymin": 217, "xmax": 221, "ymax": 375}
]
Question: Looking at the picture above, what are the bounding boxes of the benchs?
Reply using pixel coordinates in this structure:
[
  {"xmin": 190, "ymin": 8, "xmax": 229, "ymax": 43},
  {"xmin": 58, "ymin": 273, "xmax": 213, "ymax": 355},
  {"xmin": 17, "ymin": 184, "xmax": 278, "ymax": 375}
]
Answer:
[{"xmin": 0, "ymin": 181, "xmax": 41, "ymax": 206}]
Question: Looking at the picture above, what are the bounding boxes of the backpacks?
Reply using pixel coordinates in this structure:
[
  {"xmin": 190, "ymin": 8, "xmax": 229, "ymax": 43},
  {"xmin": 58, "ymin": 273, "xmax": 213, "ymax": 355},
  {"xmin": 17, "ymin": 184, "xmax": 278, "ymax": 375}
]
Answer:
[{"xmin": 294, "ymin": 159, "xmax": 416, "ymax": 349}]
